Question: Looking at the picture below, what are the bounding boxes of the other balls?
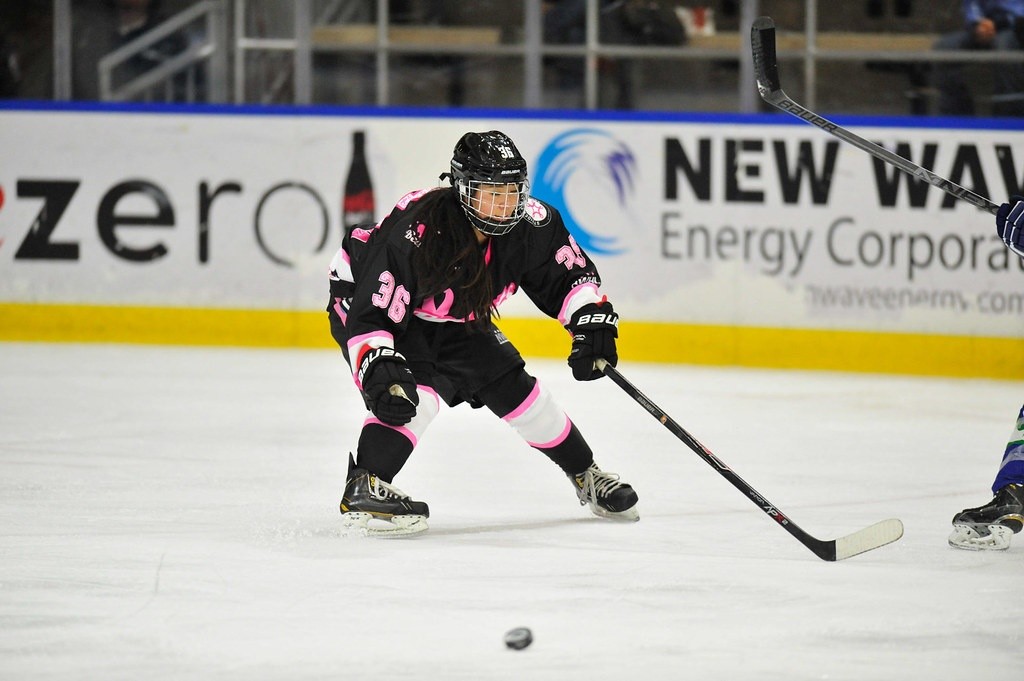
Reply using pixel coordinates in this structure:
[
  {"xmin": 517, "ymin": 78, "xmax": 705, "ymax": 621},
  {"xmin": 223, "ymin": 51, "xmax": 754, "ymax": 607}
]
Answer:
[{"xmin": 504, "ymin": 626, "xmax": 533, "ymax": 651}]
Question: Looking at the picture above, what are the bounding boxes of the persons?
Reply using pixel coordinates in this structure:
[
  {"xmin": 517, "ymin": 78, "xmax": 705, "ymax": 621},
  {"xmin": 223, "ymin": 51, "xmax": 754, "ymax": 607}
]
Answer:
[
  {"xmin": 325, "ymin": 130, "xmax": 640, "ymax": 538},
  {"xmin": 1, "ymin": 1, "xmax": 1024, "ymax": 117},
  {"xmin": 948, "ymin": 196, "xmax": 1024, "ymax": 553}
]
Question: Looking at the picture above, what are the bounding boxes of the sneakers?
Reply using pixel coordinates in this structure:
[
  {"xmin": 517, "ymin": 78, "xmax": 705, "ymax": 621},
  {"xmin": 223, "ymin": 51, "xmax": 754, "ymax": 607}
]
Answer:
[
  {"xmin": 949, "ymin": 483, "xmax": 1024, "ymax": 550},
  {"xmin": 340, "ymin": 450, "xmax": 429, "ymax": 539},
  {"xmin": 568, "ymin": 462, "xmax": 640, "ymax": 525}
]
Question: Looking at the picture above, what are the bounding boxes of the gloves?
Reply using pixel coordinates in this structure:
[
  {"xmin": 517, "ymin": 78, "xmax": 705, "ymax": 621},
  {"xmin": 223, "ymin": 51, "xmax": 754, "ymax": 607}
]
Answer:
[
  {"xmin": 564, "ymin": 303, "xmax": 617, "ymax": 382},
  {"xmin": 355, "ymin": 348, "xmax": 420, "ymax": 426}
]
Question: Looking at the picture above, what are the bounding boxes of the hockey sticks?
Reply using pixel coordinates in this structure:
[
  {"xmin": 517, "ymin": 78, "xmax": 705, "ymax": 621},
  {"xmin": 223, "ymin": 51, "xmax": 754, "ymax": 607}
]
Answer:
[
  {"xmin": 596, "ymin": 358, "xmax": 905, "ymax": 562},
  {"xmin": 749, "ymin": 16, "xmax": 1001, "ymax": 215}
]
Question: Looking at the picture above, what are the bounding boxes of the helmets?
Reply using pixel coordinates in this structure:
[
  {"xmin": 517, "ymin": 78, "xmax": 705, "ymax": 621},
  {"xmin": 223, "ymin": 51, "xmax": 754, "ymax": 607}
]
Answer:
[{"xmin": 440, "ymin": 130, "xmax": 527, "ymax": 180}]
